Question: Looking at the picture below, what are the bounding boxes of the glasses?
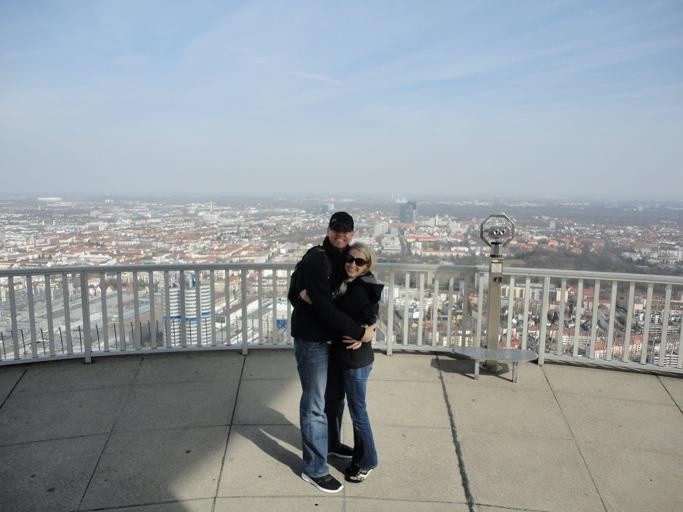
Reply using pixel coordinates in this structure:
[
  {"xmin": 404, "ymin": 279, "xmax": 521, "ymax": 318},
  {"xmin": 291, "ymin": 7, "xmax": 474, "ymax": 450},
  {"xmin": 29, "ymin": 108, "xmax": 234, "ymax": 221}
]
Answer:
[{"xmin": 345, "ymin": 254, "xmax": 367, "ymax": 266}]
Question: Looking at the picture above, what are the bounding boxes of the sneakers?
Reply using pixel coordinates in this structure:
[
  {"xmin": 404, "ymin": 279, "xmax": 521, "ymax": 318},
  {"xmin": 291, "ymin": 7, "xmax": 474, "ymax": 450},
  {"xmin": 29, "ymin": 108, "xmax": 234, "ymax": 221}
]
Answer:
[
  {"xmin": 301, "ymin": 471, "xmax": 344, "ymax": 493},
  {"xmin": 348, "ymin": 466, "xmax": 374, "ymax": 481},
  {"xmin": 327, "ymin": 444, "xmax": 354, "ymax": 459}
]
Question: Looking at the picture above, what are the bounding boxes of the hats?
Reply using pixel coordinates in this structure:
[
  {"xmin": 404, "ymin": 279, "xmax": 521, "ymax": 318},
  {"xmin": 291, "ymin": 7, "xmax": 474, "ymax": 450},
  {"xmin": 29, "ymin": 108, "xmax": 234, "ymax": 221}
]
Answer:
[{"xmin": 329, "ymin": 213, "xmax": 353, "ymax": 232}]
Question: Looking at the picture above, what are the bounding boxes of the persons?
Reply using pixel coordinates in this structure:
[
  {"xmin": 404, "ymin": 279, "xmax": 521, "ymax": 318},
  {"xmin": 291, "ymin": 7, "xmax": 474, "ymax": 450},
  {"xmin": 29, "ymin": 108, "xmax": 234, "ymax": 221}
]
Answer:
[
  {"xmin": 298, "ymin": 242, "xmax": 383, "ymax": 483},
  {"xmin": 288, "ymin": 211, "xmax": 378, "ymax": 493}
]
